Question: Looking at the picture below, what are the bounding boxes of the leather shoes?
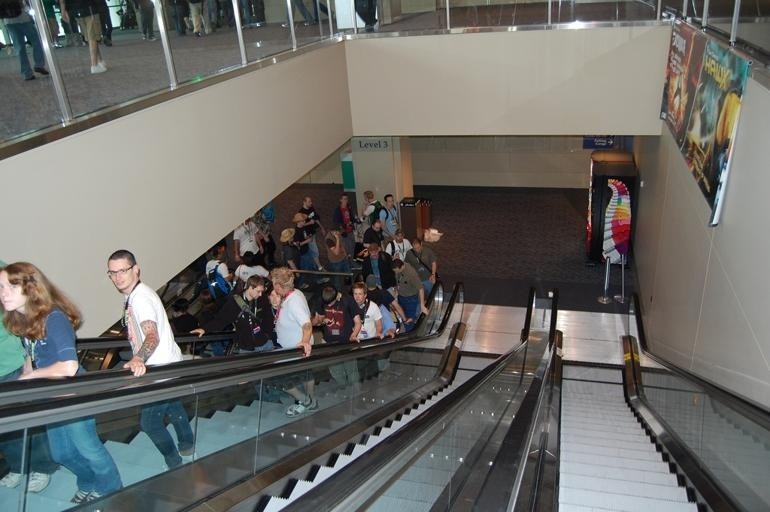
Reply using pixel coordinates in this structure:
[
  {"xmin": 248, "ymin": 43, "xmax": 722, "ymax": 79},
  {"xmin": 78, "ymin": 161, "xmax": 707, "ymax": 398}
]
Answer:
[
  {"xmin": 25, "ymin": 75, "xmax": 35, "ymax": 80},
  {"xmin": 35, "ymin": 68, "xmax": 48, "ymax": 74}
]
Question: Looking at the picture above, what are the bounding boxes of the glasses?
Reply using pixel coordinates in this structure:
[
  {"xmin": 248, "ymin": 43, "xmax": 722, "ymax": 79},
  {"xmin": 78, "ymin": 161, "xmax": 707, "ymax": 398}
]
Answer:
[{"xmin": 107, "ymin": 264, "xmax": 133, "ymax": 277}]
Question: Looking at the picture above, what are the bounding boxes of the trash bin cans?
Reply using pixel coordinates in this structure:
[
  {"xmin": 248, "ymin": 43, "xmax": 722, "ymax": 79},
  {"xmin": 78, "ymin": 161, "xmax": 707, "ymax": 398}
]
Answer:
[
  {"xmin": 340, "ymin": 148, "xmax": 355, "ymax": 192},
  {"xmin": 420, "ymin": 198, "xmax": 432, "ymax": 229},
  {"xmin": 401, "ymin": 197, "xmax": 423, "ymax": 243}
]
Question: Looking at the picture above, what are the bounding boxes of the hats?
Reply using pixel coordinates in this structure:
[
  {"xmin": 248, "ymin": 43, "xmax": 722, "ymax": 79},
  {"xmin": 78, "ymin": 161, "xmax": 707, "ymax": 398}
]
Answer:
[
  {"xmin": 291, "ymin": 213, "xmax": 307, "ymax": 222},
  {"xmin": 366, "ymin": 274, "xmax": 377, "ymax": 289},
  {"xmin": 326, "ymin": 223, "xmax": 339, "ymax": 231},
  {"xmin": 395, "ymin": 229, "xmax": 403, "ymax": 234},
  {"xmin": 280, "ymin": 228, "xmax": 295, "ymax": 242}
]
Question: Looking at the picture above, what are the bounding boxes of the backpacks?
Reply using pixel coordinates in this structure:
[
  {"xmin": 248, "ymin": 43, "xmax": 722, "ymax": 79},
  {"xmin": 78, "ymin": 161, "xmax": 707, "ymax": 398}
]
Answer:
[
  {"xmin": 207, "ymin": 262, "xmax": 231, "ymax": 301},
  {"xmin": 0, "ymin": 0, "xmax": 23, "ymax": 19},
  {"xmin": 234, "ymin": 277, "xmax": 246, "ymax": 294},
  {"xmin": 374, "ymin": 205, "xmax": 396, "ymax": 220}
]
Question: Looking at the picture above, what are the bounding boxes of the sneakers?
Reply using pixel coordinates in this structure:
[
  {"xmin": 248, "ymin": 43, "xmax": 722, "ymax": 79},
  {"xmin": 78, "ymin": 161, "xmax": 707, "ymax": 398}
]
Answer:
[
  {"xmin": 281, "ymin": 23, "xmax": 289, "ymax": 28},
  {"xmin": 317, "ymin": 266, "xmax": 328, "ymax": 272},
  {"xmin": 27, "ymin": 471, "xmax": 51, "ymax": 495},
  {"xmin": 79, "ymin": 490, "xmax": 104, "ymax": 503},
  {"xmin": 70, "ymin": 489, "xmax": 92, "ymax": 503},
  {"xmin": 163, "ymin": 452, "xmax": 197, "ymax": 468},
  {"xmin": 304, "ymin": 22, "xmax": 313, "ymax": 26},
  {"xmin": 300, "ymin": 283, "xmax": 310, "ymax": 290},
  {"xmin": 0, "ymin": 471, "xmax": 28, "ymax": 489},
  {"xmin": 104, "ymin": 35, "xmax": 112, "ymax": 46},
  {"xmin": 317, "ymin": 277, "xmax": 330, "ymax": 285},
  {"xmin": 285, "ymin": 393, "xmax": 320, "ymax": 418},
  {"xmin": 53, "ymin": 41, "xmax": 63, "ymax": 48},
  {"xmin": 91, "ymin": 60, "xmax": 108, "ymax": 73}
]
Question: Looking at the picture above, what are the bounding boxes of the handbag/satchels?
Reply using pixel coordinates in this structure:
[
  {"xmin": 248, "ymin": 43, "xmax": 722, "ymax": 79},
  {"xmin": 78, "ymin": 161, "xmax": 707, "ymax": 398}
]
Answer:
[
  {"xmin": 360, "ymin": 298, "xmax": 370, "ymax": 324},
  {"xmin": 435, "ymin": 272, "xmax": 441, "ymax": 278},
  {"xmin": 299, "ymin": 241, "xmax": 308, "ymax": 256}
]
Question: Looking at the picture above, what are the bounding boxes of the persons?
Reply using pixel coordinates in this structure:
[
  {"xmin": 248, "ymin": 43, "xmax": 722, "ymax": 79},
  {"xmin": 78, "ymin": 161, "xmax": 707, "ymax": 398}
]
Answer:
[
  {"xmin": 270, "ymin": 267, "xmax": 319, "ymax": 417},
  {"xmin": 281, "ymin": 0, "xmax": 317, "ymax": 29},
  {"xmin": 170, "ymin": 298, "xmax": 196, "ymax": 354},
  {"xmin": 107, "ymin": 249, "xmax": 196, "ymax": 471},
  {"xmin": 0, "ymin": 18, "xmax": 14, "ymax": 56},
  {"xmin": 0, "ymin": 262, "xmax": 124, "ymax": 505},
  {"xmin": 42, "ymin": 0, "xmax": 113, "ymax": 75},
  {"xmin": 194, "ymin": 191, "xmax": 444, "ymax": 406},
  {"xmin": 354, "ymin": 0, "xmax": 378, "ymax": 33},
  {"xmin": 0, "ymin": 0, "xmax": 50, "ymax": 81},
  {"xmin": 311, "ymin": 0, "xmax": 335, "ymax": 25},
  {"xmin": 0, "ymin": 260, "xmax": 58, "ymax": 494},
  {"xmin": 134, "ymin": 0, "xmax": 157, "ymax": 42},
  {"xmin": 161, "ymin": 0, "xmax": 266, "ymax": 39}
]
